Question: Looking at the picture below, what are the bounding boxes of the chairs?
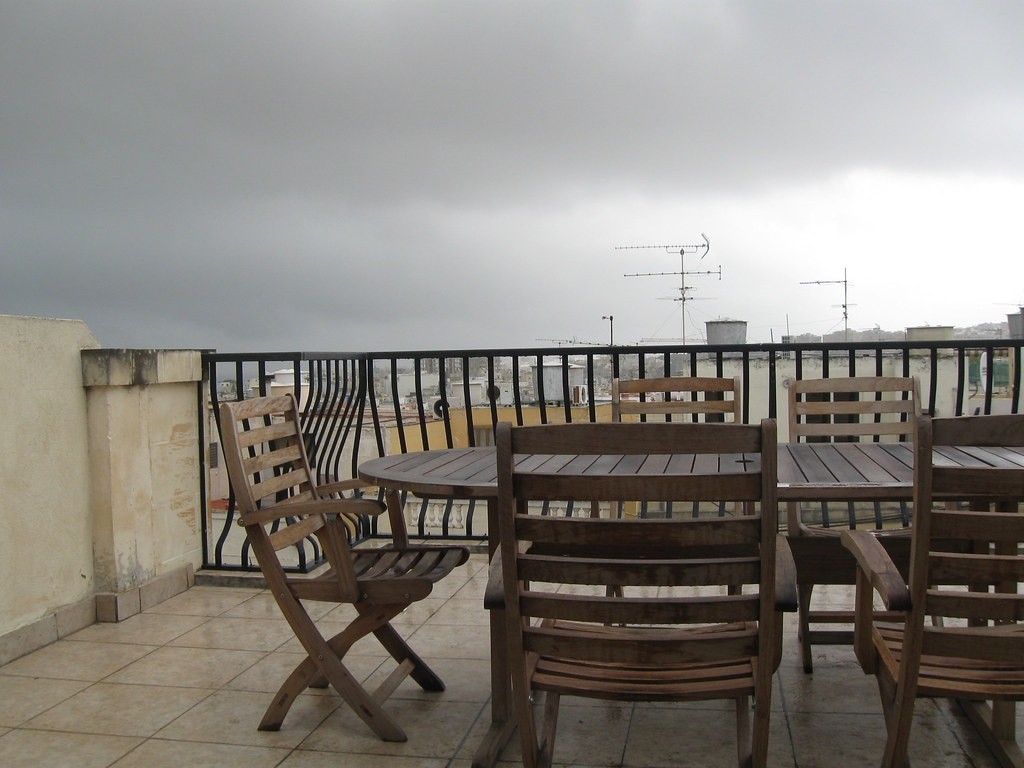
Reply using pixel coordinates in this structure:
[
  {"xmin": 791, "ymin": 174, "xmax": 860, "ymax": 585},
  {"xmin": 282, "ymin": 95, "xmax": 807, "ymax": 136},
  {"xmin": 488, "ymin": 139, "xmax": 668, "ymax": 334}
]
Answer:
[
  {"xmin": 484, "ymin": 377, "xmax": 1024, "ymax": 768},
  {"xmin": 221, "ymin": 393, "xmax": 470, "ymax": 744}
]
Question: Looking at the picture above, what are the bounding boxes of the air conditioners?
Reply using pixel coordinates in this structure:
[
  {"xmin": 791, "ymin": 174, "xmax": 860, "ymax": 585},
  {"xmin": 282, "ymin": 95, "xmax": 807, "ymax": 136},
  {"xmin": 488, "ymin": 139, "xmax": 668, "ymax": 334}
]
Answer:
[
  {"xmin": 484, "ymin": 383, "xmax": 514, "ymax": 405},
  {"xmin": 429, "ymin": 397, "xmax": 461, "ymax": 419},
  {"xmin": 782, "ymin": 335, "xmax": 797, "ymax": 359},
  {"xmin": 572, "ymin": 385, "xmax": 588, "ymax": 405}
]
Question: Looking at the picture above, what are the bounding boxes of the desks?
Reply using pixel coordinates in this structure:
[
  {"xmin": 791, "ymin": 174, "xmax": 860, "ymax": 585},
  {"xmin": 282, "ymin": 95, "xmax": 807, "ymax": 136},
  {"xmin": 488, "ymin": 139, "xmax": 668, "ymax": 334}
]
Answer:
[{"xmin": 358, "ymin": 443, "xmax": 1024, "ymax": 767}]
